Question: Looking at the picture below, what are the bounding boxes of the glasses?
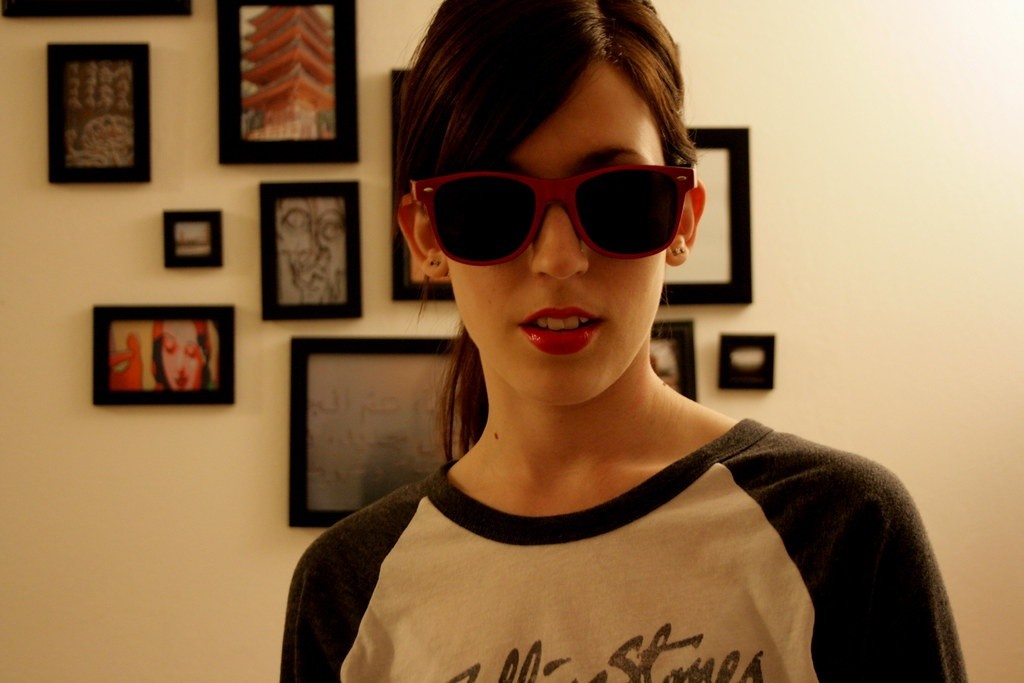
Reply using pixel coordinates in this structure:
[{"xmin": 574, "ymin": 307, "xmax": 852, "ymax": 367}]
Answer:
[{"xmin": 410, "ymin": 164, "xmax": 695, "ymax": 266}]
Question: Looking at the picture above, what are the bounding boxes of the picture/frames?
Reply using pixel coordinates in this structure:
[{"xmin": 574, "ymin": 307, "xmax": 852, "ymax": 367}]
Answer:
[
  {"xmin": 660, "ymin": 127, "xmax": 752, "ymax": 335},
  {"xmin": 390, "ymin": 68, "xmax": 455, "ymax": 302},
  {"xmin": 91, "ymin": 306, "xmax": 237, "ymax": 407},
  {"xmin": 258, "ymin": 181, "xmax": 363, "ymax": 322},
  {"xmin": 215, "ymin": 0, "xmax": 362, "ymax": 165},
  {"xmin": 288, "ymin": 333, "xmax": 462, "ymax": 529},
  {"xmin": 718, "ymin": 334, "xmax": 775, "ymax": 391},
  {"xmin": 649, "ymin": 319, "xmax": 697, "ymax": 402},
  {"xmin": 47, "ymin": 42, "xmax": 153, "ymax": 185},
  {"xmin": 163, "ymin": 210, "xmax": 224, "ymax": 269}
]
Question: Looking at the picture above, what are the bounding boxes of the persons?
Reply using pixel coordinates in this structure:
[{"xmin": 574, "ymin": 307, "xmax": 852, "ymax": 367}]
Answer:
[{"xmin": 280, "ymin": 0, "xmax": 969, "ymax": 683}]
